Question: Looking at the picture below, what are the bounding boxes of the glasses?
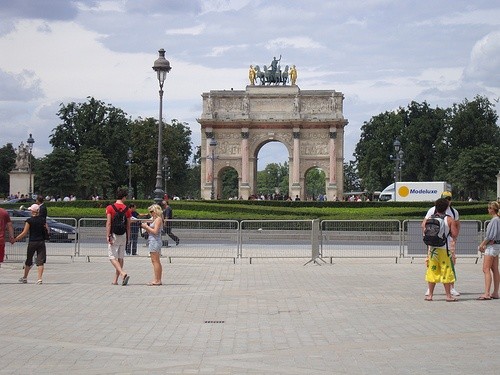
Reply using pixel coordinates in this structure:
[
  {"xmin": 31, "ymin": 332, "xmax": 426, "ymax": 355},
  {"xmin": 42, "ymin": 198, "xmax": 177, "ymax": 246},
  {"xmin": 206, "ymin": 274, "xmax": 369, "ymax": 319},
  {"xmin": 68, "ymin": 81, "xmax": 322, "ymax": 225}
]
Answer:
[{"xmin": 150, "ymin": 211, "xmax": 153, "ymax": 213}]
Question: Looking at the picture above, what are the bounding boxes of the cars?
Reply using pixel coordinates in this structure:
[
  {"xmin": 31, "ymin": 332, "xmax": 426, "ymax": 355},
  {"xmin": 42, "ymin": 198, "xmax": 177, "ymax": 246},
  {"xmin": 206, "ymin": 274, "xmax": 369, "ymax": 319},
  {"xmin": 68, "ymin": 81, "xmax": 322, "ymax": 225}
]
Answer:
[
  {"xmin": 3, "ymin": 209, "xmax": 79, "ymax": 243},
  {"xmin": 7, "ymin": 198, "xmax": 36, "ymax": 204}
]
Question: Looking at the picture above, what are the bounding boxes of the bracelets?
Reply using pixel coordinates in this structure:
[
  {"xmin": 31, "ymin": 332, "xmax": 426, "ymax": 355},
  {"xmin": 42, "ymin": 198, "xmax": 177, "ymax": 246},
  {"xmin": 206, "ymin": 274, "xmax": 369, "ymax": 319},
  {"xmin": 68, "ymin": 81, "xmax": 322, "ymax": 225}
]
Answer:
[{"xmin": 145, "ymin": 226, "xmax": 148, "ymax": 229}]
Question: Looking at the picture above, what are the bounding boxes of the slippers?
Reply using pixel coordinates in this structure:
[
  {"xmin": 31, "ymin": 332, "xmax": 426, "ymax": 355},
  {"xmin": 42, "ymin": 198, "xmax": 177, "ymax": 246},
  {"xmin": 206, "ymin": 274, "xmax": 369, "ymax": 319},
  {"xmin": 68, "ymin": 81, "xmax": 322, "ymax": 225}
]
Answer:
[
  {"xmin": 491, "ymin": 297, "xmax": 498, "ymax": 299},
  {"xmin": 478, "ymin": 296, "xmax": 491, "ymax": 300},
  {"xmin": 446, "ymin": 297, "xmax": 458, "ymax": 302},
  {"xmin": 424, "ymin": 298, "xmax": 432, "ymax": 301}
]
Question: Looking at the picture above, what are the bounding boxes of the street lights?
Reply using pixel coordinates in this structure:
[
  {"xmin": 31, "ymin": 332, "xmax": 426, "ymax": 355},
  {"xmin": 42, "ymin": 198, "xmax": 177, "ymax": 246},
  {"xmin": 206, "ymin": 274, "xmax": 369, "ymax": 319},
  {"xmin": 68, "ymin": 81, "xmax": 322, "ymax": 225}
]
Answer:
[
  {"xmin": 151, "ymin": 48, "xmax": 173, "ymax": 206},
  {"xmin": 389, "ymin": 139, "xmax": 405, "ymax": 182},
  {"xmin": 26, "ymin": 133, "xmax": 35, "ymax": 198},
  {"xmin": 163, "ymin": 155, "xmax": 169, "ymax": 193},
  {"xmin": 127, "ymin": 147, "xmax": 134, "ymax": 200}
]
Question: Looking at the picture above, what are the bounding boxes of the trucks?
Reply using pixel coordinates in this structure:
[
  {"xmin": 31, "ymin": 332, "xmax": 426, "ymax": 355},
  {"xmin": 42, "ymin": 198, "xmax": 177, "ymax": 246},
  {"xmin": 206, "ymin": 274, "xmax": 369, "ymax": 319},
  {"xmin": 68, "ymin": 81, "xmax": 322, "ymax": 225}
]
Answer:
[{"xmin": 377, "ymin": 181, "xmax": 453, "ymax": 202}]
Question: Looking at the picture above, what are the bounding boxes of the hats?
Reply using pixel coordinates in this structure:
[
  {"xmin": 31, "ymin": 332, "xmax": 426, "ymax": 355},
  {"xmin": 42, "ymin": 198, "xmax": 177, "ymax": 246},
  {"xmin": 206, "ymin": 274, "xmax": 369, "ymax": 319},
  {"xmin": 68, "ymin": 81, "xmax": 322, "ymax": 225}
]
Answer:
[
  {"xmin": 441, "ymin": 191, "xmax": 451, "ymax": 199},
  {"xmin": 28, "ymin": 204, "xmax": 39, "ymax": 212}
]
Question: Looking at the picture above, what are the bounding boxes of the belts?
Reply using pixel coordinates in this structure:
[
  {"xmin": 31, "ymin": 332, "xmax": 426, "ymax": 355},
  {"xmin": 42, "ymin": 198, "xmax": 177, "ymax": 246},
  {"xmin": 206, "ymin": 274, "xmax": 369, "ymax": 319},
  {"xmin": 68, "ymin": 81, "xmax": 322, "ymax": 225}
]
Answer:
[{"xmin": 493, "ymin": 240, "xmax": 500, "ymax": 244}]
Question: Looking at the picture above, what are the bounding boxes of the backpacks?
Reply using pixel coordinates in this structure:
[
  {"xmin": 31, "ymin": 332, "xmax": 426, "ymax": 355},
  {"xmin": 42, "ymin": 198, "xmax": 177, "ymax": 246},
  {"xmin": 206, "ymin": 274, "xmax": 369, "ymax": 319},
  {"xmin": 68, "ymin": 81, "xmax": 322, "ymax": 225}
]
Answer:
[
  {"xmin": 111, "ymin": 203, "xmax": 129, "ymax": 235},
  {"xmin": 422, "ymin": 214, "xmax": 451, "ymax": 246}
]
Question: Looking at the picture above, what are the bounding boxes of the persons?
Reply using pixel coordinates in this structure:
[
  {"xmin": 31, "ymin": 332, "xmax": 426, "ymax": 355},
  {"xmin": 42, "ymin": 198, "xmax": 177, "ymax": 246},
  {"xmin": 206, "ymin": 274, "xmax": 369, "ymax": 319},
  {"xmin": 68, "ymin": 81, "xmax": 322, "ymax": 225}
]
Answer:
[
  {"xmin": 19, "ymin": 205, "xmax": 25, "ymax": 212},
  {"xmin": 162, "ymin": 200, "xmax": 179, "ymax": 246},
  {"xmin": 22, "ymin": 195, "xmax": 48, "ymax": 230},
  {"xmin": 268, "ymin": 55, "xmax": 282, "ymax": 74},
  {"xmin": 140, "ymin": 204, "xmax": 164, "ymax": 286},
  {"xmin": 3, "ymin": 191, "xmax": 77, "ymax": 203},
  {"xmin": 229, "ymin": 192, "xmax": 301, "ymax": 202},
  {"xmin": 162, "ymin": 193, "xmax": 205, "ymax": 201},
  {"xmin": 10, "ymin": 204, "xmax": 49, "ymax": 285},
  {"xmin": 249, "ymin": 65, "xmax": 257, "ymax": 86},
  {"xmin": 477, "ymin": 201, "xmax": 500, "ymax": 300},
  {"xmin": 290, "ymin": 65, "xmax": 298, "ymax": 85},
  {"xmin": 105, "ymin": 190, "xmax": 132, "ymax": 286},
  {"xmin": 315, "ymin": 191, "xmax": 370, "ymax": 202},
  {"xmin": 125, "ymin": 202, "xmax": 140, "ymax": 255},
  {"xmin": 0, "ymin": 207, "xmax": 14, "ymax": 268},
  {"xmin": 422, "ymin": 191, "xmax": 460, "ymax": 302},
  {"xmin": 92, "ymin": 194, "xmax": 100, "ymax": 201},
  {"xmin": 468, "ymin": 197, "xmax": 473, "ymax": 202}
]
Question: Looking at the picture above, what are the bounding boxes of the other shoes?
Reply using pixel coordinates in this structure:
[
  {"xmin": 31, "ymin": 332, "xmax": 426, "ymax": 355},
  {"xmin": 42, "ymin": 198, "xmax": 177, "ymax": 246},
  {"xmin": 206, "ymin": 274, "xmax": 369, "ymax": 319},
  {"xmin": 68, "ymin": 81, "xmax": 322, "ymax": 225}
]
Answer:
[
  {"xmin": 449, "ymin": 289, "xmax": 460, "ymax": 296},
  {"xmin": 19, "ymin": 278, "xmax": 27, "ymax": 283},
  {"xmin": 176, "ymin": 238, "xmax": 180, "ymax": 245},
  {"xmin": 122, "ymin": 275, "xmax": 129, "ymax": 285},
  {"xmin": 425, "ymin": 290, "xmax": 429, "ymax": 295},
  {"xmin": 37, "ymin": 280, "xmax": 42, "ymax": 284}
]
